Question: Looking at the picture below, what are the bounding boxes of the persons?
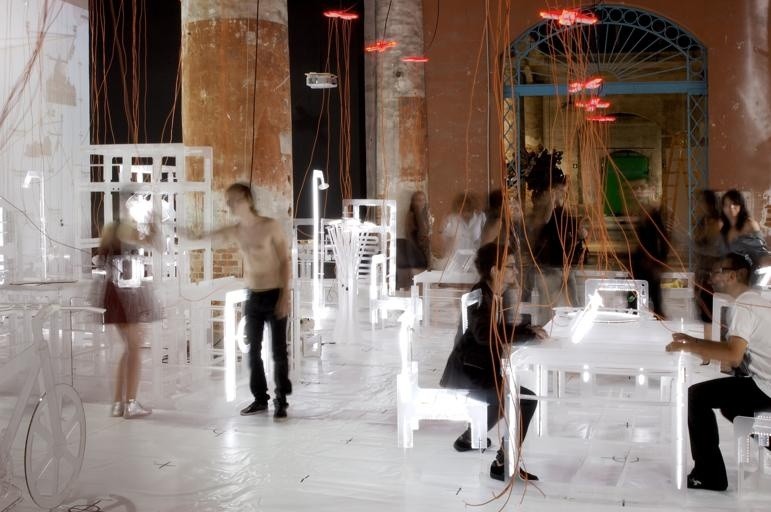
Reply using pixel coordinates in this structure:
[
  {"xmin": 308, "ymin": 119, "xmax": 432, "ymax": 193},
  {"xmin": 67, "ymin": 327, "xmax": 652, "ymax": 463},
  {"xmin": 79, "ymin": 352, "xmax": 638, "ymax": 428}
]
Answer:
[
  {"xmin": 404, "ymin": 176, "xmax": 771, "ymax": 367},
  {"xmin": 665, "ymin": 252, "xmax": 771, "ymax": 491},
  {"xmin": 438, "ymin": 243, "xmax": 549, "ymax": 481},
  {"xmin": 98, "ymin": 191, "xmax": 163, "ymax": 420},
  {"xmin": 176, "ymin": 182, "xmax": 292, "ymax": 423}
]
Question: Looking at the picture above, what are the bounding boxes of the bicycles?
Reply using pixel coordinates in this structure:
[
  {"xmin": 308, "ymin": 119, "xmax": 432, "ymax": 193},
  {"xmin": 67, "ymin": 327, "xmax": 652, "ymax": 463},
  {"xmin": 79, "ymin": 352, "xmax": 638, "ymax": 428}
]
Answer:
[{"xmin": 1, "ymin": 301, "xmax": 105, "ymax": 510}]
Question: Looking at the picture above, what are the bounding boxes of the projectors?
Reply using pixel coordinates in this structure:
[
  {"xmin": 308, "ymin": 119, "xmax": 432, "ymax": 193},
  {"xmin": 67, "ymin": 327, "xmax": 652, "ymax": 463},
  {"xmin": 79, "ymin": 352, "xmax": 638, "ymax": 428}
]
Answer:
[{"xmin": 306, "ymin": 72, "xmax": 338, "ymax": 88}]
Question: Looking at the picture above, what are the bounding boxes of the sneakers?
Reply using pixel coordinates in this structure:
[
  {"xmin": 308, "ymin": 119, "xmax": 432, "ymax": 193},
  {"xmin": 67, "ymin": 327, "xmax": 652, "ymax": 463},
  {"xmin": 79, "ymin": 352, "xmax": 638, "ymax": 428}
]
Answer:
[
  {"xmin": 240, "ymin": 401, "xmax": 268, "ymax": 416},
  {"xmin": 453, "ymin": 435, "xmax": 491, "ymax": 451},
  {"xmin": 687, "ymin": 474, "xmax": 728, "ymax": 491},
  {"xmin": 490, "ymin": 460, "xmax": 538, "ymax": 481},
  {"xmin": 273, "ymin": 408, "xmax": 287, "ymax": 422},
  {"xmin": 113, "ymin": 400, "xmax": 152, "ymax": 418}
]
